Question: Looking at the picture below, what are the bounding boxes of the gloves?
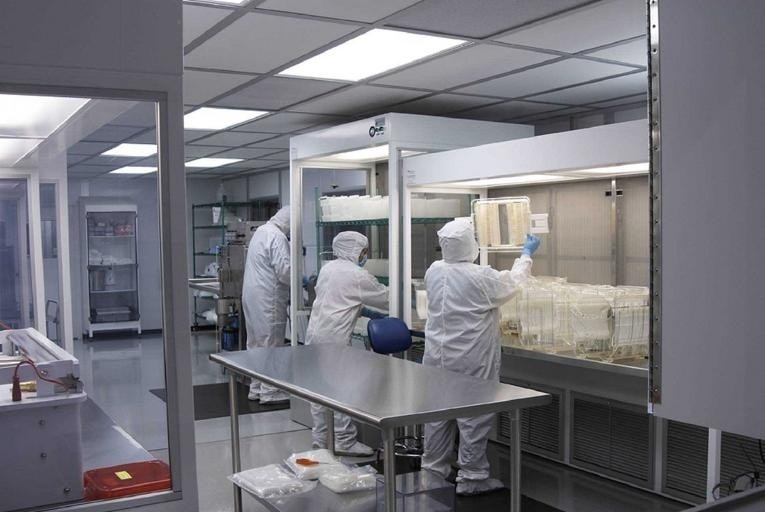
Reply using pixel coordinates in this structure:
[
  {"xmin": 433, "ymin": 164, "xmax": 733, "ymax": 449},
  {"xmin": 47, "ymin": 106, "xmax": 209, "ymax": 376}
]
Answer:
[
  {"xmin": 521, "ymin": 233, "xmax": 540, "ymax": 256},
  {"xmin": 303, "ymin": 276, "xmax": 309, "ymax": 285}
]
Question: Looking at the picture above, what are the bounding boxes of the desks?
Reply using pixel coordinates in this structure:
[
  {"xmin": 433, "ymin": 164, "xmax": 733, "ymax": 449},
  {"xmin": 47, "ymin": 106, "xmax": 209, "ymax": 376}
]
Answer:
[{"xmin": 209, "ymin": 341, "xmax": 555, "ymax": 511}]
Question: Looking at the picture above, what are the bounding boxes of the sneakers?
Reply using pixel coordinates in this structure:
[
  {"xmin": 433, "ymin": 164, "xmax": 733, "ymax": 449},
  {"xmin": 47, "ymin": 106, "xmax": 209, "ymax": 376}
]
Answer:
[
  {"xmin": 248, "ymin": 393, "xmax": 289, "ymax": 404},
  {"xmin": 445, "ymin": 469, "xmax": 505, "ymax": 495},
  {"xmin": 312, "ymin": 441, "xmax": 375, "ymax": 457}
]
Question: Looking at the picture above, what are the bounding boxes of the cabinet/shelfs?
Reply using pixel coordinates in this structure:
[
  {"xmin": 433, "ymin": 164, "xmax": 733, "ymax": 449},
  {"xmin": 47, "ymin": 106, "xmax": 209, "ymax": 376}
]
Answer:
[
  {"xmin": 191, "ymin": 193, "xmax": 273, "ymax": 332},
  {"xmin": 82, "ymin": 204, "xmax": 141, "ymax": 342}
]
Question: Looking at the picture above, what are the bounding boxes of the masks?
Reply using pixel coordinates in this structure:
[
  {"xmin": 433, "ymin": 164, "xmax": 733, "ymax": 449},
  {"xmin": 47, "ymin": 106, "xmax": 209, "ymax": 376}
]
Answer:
[{"xmin": 359, "ymin": 255, "xmax": 367, "ymax": 267}]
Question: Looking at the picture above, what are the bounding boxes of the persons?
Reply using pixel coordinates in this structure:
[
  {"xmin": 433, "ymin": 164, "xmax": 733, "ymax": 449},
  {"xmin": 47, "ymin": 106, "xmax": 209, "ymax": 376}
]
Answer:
[
  {"xmin": 304, "ymin": 231, "xmax": 416, "ymax": 456},
  {"xmin": 241, "ymin": 204, "xmax": 291, "ymax": 404},
  {"xmin": 420, "ymin": 217, "xmax": 541, "ymax": 496}
]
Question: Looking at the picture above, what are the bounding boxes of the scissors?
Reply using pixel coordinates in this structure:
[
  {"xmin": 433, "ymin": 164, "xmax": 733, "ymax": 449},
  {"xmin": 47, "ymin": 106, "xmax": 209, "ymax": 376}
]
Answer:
[{"xmin": 296, "ymin": 459, "xmax": 330, "ymax": 466}]
